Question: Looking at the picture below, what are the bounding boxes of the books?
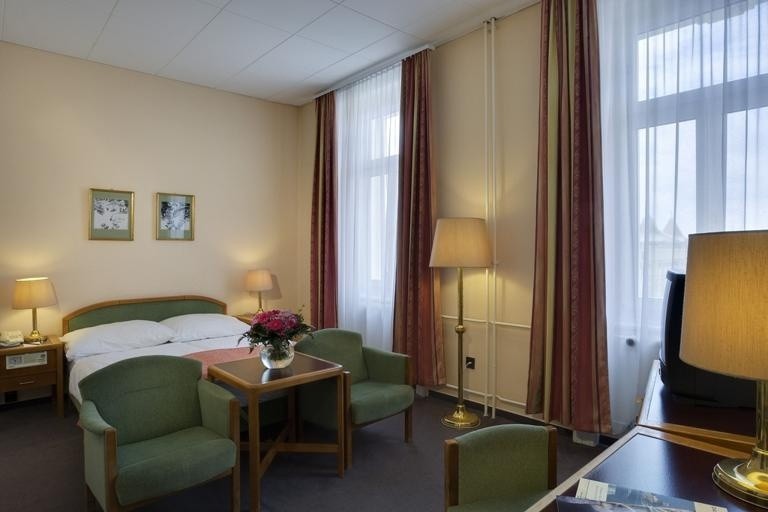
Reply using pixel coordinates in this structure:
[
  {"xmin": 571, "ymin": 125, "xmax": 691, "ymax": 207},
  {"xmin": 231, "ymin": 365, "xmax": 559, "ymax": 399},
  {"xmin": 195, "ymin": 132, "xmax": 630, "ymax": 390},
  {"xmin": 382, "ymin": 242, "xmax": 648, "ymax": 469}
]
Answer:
[
  {"xmin": 556, "ymin": 495, "xmax": 690, "ymax": 512},
  {"xmin": 575, "ymin": 478, "xmax": 727, "ymax": 512}
]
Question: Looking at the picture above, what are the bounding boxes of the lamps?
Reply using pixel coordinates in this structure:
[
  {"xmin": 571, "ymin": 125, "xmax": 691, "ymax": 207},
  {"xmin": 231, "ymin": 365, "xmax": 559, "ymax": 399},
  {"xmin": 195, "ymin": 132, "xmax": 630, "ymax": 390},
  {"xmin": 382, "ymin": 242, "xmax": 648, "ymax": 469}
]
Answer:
[
  {"xmin": 678, "ymin": 230, "xmax": 767, "ymax": 508},
  {"xmin": 428, "ymin": 217, "xmax": 493, "ymax": 429},
  {"xmin": 12, "ymin": 277, "xmax": 58, "ymax": 342},
  {"xmin": 246, "ymin": 269, "xmax": 274, "ymax": 318}
]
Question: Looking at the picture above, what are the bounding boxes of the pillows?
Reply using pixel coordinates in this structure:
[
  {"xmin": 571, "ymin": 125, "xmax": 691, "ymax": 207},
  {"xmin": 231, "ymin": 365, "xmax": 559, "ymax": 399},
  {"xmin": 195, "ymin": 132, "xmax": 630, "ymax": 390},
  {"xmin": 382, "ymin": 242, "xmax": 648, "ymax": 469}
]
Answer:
[
  {"xmin": 59, "ymin": 320, "xmax": 179, "ymax": 361},
  {"xmin": 158, "ymin": 313, "xmax": 251, "ymax": 343}
]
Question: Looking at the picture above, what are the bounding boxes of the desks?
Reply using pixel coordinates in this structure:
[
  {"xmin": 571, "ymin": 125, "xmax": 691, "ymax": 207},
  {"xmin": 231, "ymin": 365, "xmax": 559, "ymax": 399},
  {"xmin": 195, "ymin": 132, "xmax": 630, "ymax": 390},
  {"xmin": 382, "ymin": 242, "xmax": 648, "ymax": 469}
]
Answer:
[
  {"xmin": 524, "ymin": 427, "xmax": 768, "ymax": 512},
  {"xmin": 637, "ymin": 360, "xmax": 757, "ymax": 445}
]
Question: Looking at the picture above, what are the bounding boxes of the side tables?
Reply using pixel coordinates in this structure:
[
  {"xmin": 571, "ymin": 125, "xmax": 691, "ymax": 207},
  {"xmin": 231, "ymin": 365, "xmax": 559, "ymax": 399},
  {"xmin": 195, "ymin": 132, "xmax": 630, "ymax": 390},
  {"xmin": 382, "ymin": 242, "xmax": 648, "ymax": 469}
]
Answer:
[{"xmin": 207, "ymin": 351, "xmax": 345, "ymax": 510}]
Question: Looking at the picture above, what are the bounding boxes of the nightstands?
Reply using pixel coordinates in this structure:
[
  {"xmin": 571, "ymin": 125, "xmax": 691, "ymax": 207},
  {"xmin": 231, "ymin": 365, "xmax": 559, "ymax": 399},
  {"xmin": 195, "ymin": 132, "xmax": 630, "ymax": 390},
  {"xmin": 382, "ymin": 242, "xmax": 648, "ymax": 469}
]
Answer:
[{"xmin": 0, "ymin": 336, "xmax": 63, "ymax": 414}]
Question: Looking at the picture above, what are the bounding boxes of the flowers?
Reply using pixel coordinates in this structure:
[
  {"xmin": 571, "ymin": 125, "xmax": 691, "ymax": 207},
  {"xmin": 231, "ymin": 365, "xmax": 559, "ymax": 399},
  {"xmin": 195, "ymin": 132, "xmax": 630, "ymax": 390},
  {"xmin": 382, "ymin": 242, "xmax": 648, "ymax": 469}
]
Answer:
[{"xmin": 237, "ymin": 310, "xmax": 316, "ymax": 360}]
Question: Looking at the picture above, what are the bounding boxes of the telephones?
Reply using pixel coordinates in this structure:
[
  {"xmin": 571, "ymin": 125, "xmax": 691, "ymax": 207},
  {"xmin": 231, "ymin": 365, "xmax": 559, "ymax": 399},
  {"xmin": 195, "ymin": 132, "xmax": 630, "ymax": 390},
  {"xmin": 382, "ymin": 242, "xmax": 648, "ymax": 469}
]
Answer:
[{"xmin": 0, "ymin": 329, "xmax": 24, "ymax": 348}]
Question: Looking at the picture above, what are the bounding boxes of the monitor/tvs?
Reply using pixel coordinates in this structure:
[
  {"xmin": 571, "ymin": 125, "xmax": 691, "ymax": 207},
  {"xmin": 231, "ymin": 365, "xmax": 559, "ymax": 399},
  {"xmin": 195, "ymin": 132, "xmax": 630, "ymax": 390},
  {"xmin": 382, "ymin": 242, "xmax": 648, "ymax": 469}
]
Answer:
[{"xmin": 660, "ymin": 269, "xmax": 757, "ymax": 405}]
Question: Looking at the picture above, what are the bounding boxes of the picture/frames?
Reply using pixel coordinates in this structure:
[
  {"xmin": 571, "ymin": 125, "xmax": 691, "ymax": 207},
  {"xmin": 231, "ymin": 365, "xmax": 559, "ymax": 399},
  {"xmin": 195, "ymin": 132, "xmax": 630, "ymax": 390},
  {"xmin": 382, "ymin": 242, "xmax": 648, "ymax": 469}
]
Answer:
[
  {"xmin": 87, "ymin": 187, "xmax": 135, "ymax": 242},
  {"xmin": 153, "ymin": 191, "xmax": 195, "ymax": 242}
]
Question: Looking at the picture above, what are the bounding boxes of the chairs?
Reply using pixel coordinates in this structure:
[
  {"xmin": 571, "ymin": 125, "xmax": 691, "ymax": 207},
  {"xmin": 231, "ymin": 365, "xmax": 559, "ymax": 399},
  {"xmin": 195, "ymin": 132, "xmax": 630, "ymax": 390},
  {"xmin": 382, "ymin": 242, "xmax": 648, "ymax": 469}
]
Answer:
[
  {"xmin": 77, "ymin": 355, "xmax": 241, "ymax": 510},
  {"xmin": 294, "ymin": 328, "xmax": 415, "ymax": 469},
  {"xmin": 442, "ymin": 425, "xmax": 558, "ymax": 511}
]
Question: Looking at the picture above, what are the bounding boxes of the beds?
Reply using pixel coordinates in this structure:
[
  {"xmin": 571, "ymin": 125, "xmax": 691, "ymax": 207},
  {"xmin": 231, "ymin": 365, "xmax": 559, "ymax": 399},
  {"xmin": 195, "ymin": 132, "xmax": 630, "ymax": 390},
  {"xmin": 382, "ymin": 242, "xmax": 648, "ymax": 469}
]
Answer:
[{"xmin": 62, "ymin": 295, "xmax": 297, "ymax": 414}]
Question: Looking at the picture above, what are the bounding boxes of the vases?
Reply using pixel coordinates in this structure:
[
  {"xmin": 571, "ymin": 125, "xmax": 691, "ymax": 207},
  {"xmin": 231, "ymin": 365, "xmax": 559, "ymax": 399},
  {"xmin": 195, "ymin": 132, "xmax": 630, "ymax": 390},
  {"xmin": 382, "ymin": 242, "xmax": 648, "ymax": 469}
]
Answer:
[{"xmin": 260, "ymin": 342, "xmax": 294, "ymax": 369}]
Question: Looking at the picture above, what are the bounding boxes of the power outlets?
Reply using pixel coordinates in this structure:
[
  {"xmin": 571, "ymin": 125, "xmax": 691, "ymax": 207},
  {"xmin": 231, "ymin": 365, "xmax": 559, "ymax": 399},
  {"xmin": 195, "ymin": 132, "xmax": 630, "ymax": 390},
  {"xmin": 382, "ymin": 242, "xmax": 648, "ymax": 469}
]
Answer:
[{"xmin": 466, "ymin": 356, "xmax": 475, "ymax": 369}]
